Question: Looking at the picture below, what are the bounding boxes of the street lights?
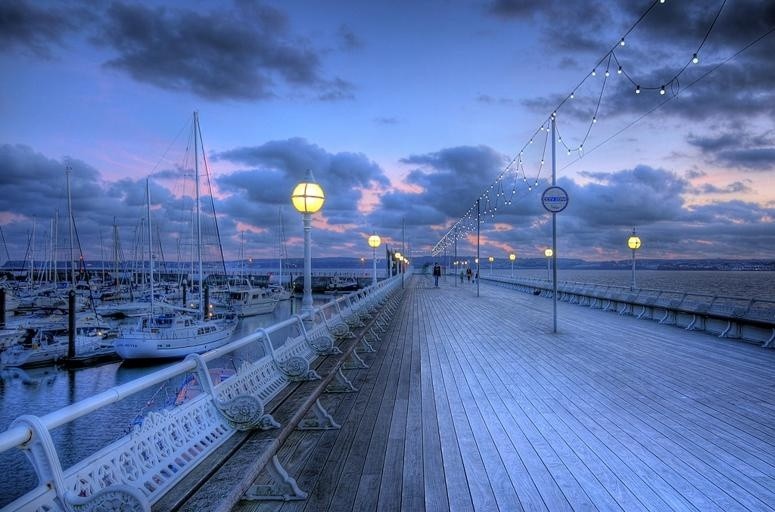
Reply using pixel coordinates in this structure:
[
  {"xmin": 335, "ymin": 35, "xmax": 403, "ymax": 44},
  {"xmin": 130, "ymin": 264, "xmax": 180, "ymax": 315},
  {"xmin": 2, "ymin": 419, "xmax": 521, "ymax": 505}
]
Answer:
[
  {"xmin": 628, "ymin": 224, "xmax": 642, "ymax": 291},
  {"xmin": 392, "ymin": 249, "xmax": 410, "ymax": 275},
  {"xmin": 453, "ymin": 245, "xmax": 556, "ymax": 285}
]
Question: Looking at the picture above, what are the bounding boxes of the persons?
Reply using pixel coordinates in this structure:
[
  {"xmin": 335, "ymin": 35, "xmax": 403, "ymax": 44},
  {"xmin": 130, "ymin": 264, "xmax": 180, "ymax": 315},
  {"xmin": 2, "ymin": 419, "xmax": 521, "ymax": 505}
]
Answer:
[
  {"xmin": 467, "ymin": 266, "xmax": 471, "ymax": 281},
  {"xmin": 460, "ymin": 271, "xmax": 464, "ymax": 283},
  {"xmin": 433, "ymin": 262, "xmax": 440, "ymax": 287}
]
